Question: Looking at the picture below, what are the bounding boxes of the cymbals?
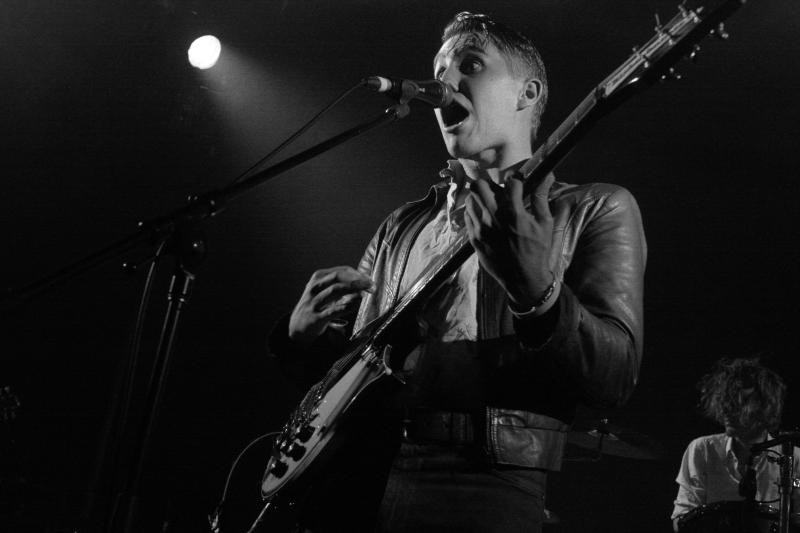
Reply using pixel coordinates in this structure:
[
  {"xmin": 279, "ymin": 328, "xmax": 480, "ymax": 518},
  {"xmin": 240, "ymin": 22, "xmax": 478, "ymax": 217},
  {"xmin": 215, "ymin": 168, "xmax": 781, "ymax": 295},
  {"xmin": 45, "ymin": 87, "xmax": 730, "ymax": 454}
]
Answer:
[{"xmin": 565, "ymin": 430, "xmax": 657, "ymax": 461}]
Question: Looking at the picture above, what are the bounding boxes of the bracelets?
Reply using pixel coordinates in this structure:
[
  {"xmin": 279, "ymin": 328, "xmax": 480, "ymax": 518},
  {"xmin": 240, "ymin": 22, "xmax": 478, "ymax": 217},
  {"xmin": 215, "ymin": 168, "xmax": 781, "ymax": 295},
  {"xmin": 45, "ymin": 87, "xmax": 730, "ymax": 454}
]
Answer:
[{"xmin": 508, "ymin": 271, "xmax": 556, "ymax": 316}]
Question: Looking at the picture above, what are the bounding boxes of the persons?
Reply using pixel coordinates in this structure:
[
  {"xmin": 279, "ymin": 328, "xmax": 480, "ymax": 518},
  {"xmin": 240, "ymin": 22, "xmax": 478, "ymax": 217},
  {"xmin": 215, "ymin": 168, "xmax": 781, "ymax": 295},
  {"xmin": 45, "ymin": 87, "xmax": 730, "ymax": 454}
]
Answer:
[
  {"xmin": 265, "ymin": 11, "xmax": 647, "ymax": 533},
  {"xmin": 671, "ymin": 360, "xmax": 800, "ymax": 533}
]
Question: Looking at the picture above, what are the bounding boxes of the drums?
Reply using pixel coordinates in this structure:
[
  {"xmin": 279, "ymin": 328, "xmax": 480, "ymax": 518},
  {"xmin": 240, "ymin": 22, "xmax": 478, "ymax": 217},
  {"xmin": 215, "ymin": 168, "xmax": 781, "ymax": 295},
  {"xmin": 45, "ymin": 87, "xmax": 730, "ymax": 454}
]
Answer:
[{"xmin": 677, "ymin": 499, "xmax": 781, "ymax": 533}]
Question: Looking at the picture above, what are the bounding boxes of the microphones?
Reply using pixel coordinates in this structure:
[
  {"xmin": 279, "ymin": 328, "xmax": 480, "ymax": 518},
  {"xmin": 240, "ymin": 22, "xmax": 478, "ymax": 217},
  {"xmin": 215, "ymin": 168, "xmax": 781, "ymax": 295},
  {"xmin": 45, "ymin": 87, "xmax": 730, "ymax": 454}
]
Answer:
[{"xmin": 362, "ymin": 76, "xmax": 455, "ymax": 108}]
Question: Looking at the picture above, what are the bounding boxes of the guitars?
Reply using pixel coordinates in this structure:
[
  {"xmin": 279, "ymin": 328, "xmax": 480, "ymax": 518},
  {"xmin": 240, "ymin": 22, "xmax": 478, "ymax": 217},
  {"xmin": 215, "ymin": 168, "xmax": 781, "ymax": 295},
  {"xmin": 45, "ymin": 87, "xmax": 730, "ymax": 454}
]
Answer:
[{"xmin": 258, "ymin": 0, "xmax": 746, "ymax": 508}]
